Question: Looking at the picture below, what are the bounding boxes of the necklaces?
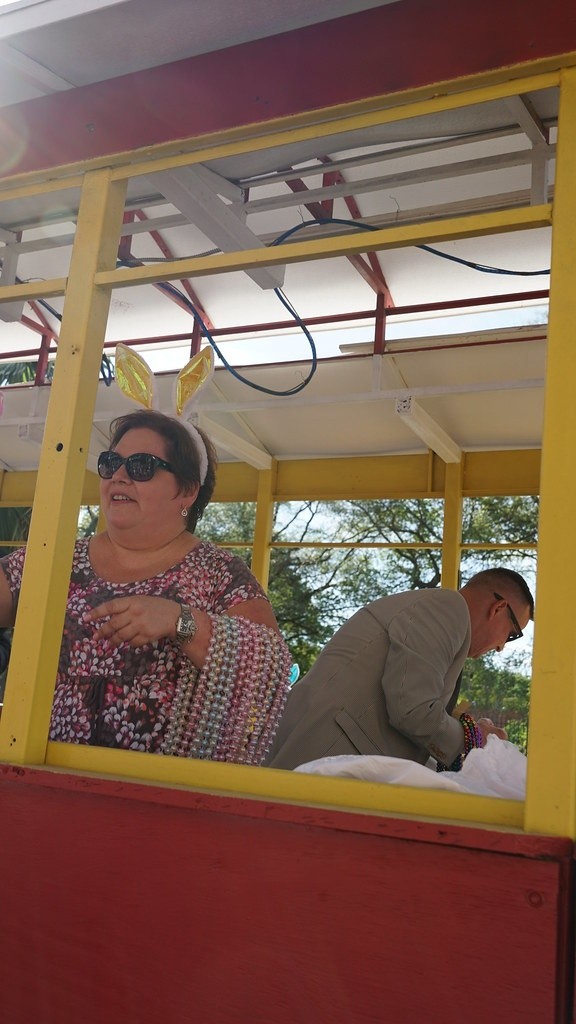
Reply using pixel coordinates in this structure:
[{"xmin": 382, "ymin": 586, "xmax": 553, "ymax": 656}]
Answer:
[
  {"xmin": 437, "ymin": 713, "xmax": 482, "ymax": 773},
  {"xmin": 156, "ymin": 610, "xmax": 293, "ymax": 767}
]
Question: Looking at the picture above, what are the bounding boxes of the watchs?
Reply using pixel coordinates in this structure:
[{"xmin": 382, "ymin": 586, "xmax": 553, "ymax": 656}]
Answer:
[{"xmin": 169, "ymin": 603, "xmax": 195, "ymax": 647}]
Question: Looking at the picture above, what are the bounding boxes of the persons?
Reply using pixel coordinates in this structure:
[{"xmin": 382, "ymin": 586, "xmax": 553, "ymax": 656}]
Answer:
[
  {"xmin": 0, "ymin": 409, "xmax": 280, "ymax": 753},
  {"xmin": 264, "ymin": 567, "xmax": 534, "ymax": 772}
]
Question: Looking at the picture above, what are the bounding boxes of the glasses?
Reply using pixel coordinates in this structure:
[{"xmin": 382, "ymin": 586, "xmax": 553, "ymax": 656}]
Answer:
[
  {"xmin": 493, "ymin": 591, "xmax": 523, "ymax": 643},
  {"xmin": 98, "ymin": 451, "xmax": 175, "ymax": 482}
]
